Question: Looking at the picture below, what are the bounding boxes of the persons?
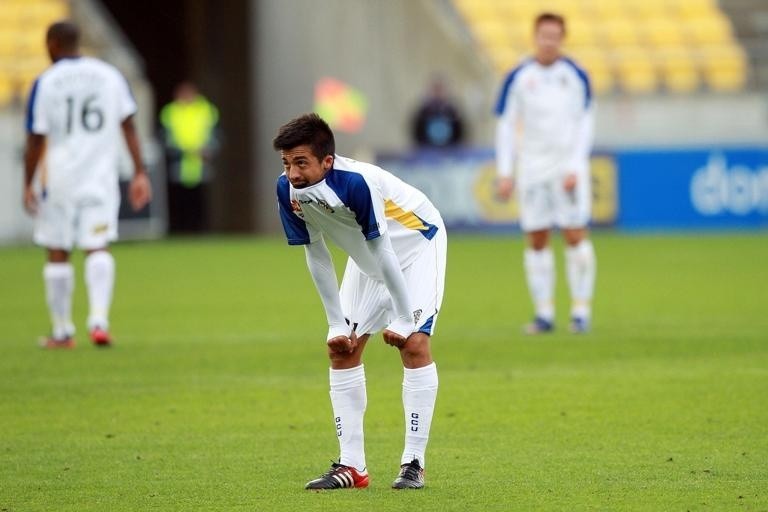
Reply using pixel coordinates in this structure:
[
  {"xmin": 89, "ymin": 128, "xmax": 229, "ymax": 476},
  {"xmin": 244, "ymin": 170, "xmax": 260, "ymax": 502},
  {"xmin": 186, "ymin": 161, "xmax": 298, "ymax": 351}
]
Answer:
[
  {"xmin": 19, "ymin": 18, "xmax": 154, "ymax": 352},
  {"xmin": 274, "ymin": 112, "xmax": 448, "ymax": 492},
  {"xmin": 411, "ymin": 77, "xmax": 464, "ymax": 152},
  {"xmin": 491, "ymin": 12, "xmax": 596, "ymax": 336},
  {"xmin": 157, "ymin": 80, "xmax": 222, "ymax": 235}
]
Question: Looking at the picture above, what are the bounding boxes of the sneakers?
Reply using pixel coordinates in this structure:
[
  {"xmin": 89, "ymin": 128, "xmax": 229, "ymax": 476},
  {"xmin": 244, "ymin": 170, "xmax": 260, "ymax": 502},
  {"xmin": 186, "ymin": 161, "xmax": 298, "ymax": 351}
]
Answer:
[
  {"xmin": 89, "ymin": 326, "xmax": 112, "ymax": 345},
  {"xmin": 41, "ymin": 333, "xmax": 75, "ymax": 349},
  {"xmin": 392, "ymin": 462, "xmax": 425, "ymax": 489},
  {"xmin": 526, "ymin": 316, "xmax": 589, "ymax": 334},
  {"xmin": 305, "ymin": 460, "xmax": 369, "ymax": 490}
]
